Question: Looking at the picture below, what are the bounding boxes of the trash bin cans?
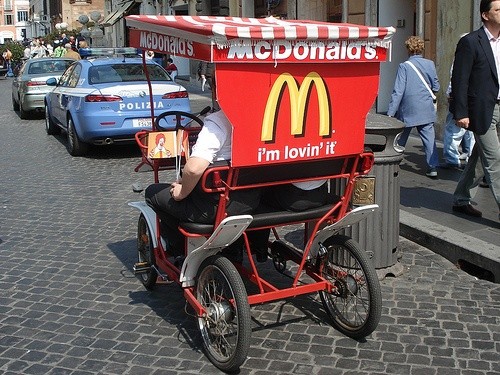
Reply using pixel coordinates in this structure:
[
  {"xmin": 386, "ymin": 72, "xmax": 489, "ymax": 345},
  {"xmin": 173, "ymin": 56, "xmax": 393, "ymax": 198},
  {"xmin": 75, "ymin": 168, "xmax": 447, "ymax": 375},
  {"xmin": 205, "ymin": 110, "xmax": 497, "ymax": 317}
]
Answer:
[{"xmin": 309, "ymin": 113, "xmax": 405, "ymax": 269}]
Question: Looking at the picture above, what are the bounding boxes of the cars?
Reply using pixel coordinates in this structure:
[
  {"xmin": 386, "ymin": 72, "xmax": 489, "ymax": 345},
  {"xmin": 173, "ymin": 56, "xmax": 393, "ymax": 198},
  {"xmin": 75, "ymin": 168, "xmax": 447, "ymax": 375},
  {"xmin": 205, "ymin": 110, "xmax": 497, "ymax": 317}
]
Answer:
[
  {"xmin": 44, "ymin": 47, "xmax": 193, "ymax": 157},
  {"xmin": 12, "ymin": 57, "xmax": 74, "ymax": 120}
]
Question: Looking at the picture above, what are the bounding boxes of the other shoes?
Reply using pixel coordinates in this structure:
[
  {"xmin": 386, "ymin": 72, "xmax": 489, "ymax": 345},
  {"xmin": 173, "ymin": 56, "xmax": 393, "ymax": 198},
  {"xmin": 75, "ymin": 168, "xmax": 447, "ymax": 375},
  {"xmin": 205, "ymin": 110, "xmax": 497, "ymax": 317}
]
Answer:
[
  {"xmin": 426, "ymin": 169, "xmax": 438, "ymax": 176},
  {"xmin": 439, "ymin": 163, "xmax": 458, "ymax": 169},
  {"xmin": 452, "ymin": 205, "xmax": 482, "ymax": 217}
]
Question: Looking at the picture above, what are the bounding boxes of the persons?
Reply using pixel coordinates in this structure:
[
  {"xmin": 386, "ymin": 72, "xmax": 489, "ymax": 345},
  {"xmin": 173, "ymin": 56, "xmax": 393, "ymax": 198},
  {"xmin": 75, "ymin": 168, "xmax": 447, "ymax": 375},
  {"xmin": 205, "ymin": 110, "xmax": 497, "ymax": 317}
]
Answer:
[
  {"xmin": 448, "ymin": 0, "xmax": 500, "ymax": 221},
  {"xmin": 23, "ymin": 32, "xmax": 93, "ymax": 72},
  {"xmin": 144, "ymin": 69, "xmax": 328, "ymax": 299},
  {"xmin": 164, "ymin": 58, "xmax": 178, "ymax": 82},
  {"xmin": 199, "ymin": 62, "xmax": 212, "ymax": 93},
  {"xmin": 440, "ymin": 32, "xmax": 476, "ymax": 169},
  {"xmin": 145, "ymin": 50, "xmax": 155, "ymax": 60},
  {"xmin": 2, "ymin": 47, "xmax": 13, "ymax": 77},
  {"xmin": 386, "ymin": 35, "xmax": 442, "ymax": 176}
]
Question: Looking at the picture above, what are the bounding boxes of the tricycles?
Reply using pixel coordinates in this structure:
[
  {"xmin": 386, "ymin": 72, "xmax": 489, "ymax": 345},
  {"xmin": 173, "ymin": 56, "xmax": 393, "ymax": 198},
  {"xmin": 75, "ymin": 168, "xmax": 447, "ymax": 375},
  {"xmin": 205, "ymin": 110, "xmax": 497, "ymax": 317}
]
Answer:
[{"xmin": 124, "ymin": 13, "xmax": 397, "ymax": 370}]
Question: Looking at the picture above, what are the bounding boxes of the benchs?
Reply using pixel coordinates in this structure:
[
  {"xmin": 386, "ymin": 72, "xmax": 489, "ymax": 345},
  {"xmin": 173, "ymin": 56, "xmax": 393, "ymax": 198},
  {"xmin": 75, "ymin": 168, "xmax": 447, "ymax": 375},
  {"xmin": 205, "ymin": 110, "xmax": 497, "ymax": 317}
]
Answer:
[
  {"xmin": 31, "ymin": 66, "xmax": 68, "ymax": 73},
  {"xmin": 166, "ymin": 147, "xmax": 375, "ymax": 237}
]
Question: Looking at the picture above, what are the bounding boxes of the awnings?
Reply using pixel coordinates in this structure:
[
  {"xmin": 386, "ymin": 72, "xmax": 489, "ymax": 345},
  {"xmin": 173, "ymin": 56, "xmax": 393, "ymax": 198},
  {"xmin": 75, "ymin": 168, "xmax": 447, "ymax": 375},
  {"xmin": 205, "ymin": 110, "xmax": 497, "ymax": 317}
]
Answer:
[{"xmin": 100, "ymin": 0, "xmax": 136, "ymax": 27}]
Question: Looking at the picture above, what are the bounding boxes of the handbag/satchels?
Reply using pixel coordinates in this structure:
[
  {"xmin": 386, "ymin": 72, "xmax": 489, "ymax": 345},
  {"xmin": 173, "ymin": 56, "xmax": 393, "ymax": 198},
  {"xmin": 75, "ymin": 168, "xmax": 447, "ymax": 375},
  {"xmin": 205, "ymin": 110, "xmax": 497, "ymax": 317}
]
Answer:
[{"xmin": 432, "ymin": 97, "xmax": 438, "ymax": 112}]
